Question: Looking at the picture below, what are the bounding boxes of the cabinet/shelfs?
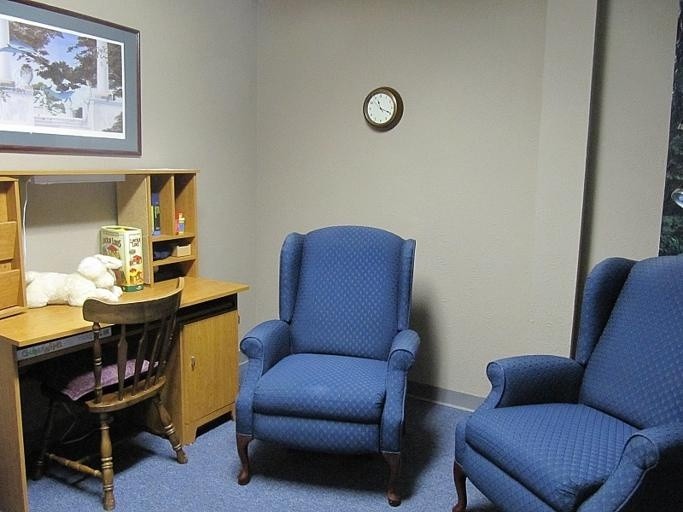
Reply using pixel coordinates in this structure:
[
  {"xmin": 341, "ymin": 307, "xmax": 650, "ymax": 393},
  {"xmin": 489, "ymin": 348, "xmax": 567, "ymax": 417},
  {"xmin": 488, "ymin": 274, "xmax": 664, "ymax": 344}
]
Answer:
[
  {"xmin": 0, "ymin": 169, "xmax": 199, "ymax": 320},
  {"xmin": 141, "ymin": 292, "xmax": 238, "ymax": 444}
]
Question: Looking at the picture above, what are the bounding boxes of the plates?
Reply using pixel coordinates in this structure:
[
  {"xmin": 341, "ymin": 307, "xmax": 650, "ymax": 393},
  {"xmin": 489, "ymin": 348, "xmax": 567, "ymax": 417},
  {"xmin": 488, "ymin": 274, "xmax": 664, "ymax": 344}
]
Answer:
[{"xmin": 152, "ymin": 247, "xmax": 172, "ymax": 260}]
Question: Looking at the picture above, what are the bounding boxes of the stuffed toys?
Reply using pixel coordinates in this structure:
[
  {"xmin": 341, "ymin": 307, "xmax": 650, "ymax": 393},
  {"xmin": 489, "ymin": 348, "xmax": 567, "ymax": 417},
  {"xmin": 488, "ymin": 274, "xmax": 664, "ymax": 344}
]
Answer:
[{"xmin": 24, "ymin": 254, "xmax": 124, "ymax": 308}]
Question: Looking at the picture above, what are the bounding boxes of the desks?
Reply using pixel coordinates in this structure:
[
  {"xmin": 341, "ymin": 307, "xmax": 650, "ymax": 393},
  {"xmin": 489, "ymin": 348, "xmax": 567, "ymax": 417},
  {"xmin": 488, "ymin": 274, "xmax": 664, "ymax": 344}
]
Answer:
[{"xmin": 0, "ymin": 281, "xmax": 249, "ymax": 512}]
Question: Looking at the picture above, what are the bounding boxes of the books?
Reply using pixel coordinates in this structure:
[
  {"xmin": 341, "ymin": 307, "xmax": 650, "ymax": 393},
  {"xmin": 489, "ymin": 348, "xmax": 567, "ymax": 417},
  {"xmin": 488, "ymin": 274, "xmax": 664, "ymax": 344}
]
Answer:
[{"xmin": 150, "ymin": 192, "xmax": 160, "ymax": 237}]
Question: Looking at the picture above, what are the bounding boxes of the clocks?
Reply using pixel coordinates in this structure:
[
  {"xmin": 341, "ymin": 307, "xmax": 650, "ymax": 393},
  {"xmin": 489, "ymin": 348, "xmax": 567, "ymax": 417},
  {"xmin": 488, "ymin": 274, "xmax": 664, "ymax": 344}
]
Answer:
[{"xmin": 362, "ymin": 87, "xmax": 404, "ymax": 132}]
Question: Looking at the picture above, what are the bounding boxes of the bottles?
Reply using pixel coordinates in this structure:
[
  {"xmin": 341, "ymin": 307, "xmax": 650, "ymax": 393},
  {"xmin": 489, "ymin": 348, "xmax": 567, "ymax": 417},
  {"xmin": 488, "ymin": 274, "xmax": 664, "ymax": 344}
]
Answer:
[
  {"xmin": 150, "ymin": 193, "xmax": 162, "ymax": 235},
  {"xmin": 176, "ymin": 213, "xmax": 186, "ymax": 233}
]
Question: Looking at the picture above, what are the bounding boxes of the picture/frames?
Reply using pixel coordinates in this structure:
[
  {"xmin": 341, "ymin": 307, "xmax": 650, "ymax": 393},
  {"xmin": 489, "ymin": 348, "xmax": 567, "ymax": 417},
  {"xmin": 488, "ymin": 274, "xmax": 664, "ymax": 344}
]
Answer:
[{"xmin": 0, "ymin": 1, "xmax": 141, "ymax": 158}]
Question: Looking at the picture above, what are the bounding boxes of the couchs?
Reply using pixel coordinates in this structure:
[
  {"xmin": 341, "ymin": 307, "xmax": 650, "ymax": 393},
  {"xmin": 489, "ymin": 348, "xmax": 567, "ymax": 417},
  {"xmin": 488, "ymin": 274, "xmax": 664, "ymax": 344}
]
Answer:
[
  {"xmin": 452, "ymin": 254, "xmax": 680, "ymax": 511},
  {"xmin": 235, "ymin": 226, "xmax": 420, "ymax": 504}
]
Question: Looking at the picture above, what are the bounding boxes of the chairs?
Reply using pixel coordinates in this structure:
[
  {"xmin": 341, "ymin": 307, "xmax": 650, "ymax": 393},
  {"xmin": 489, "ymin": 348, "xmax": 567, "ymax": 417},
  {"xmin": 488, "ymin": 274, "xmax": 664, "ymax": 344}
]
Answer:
[{"xmin": 30, "ymin": 276, "xmax": 187, "ymax": 512}]
